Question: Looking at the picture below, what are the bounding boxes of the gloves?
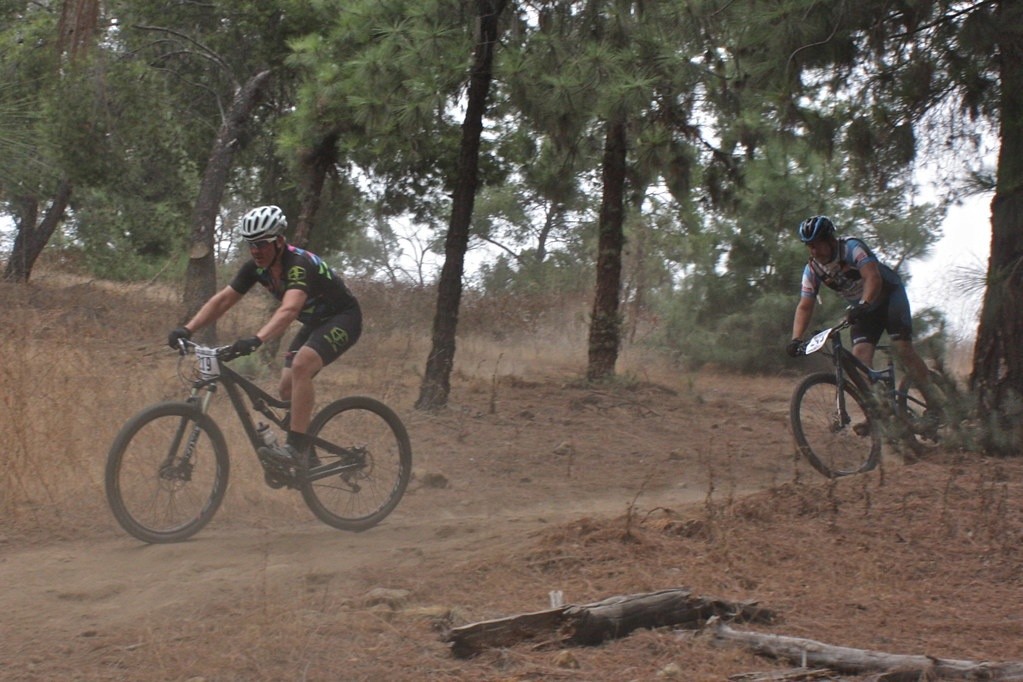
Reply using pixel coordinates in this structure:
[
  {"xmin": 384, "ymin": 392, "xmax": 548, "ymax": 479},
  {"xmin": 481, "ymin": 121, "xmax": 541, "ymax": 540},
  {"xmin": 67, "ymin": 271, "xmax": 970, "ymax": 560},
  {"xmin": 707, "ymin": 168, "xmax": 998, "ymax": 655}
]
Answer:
[
  {"xmin": 846, "ymin": 300, "xmax": 871, "ymax": 325},
  {"xmin": 228, "ymin": 335, "xmax": 262, "ymax": 358},
  {"xmin": 169, "ymin": 326, "xmax": 192, "ymax": 349},
  {"xmin": 786, "ymin": 338, "xmax": 803, "ymax": 357}
]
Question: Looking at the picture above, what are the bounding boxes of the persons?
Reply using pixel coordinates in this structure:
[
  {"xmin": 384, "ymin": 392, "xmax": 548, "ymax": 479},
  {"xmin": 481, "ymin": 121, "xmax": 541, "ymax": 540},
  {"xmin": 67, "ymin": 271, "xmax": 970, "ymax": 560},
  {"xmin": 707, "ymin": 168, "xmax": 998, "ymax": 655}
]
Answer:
[
  {"xmin": 169, "ymin": 205, "xmax": 363, "ymax": 489},
  {"xmin": 785, "ymin": 215, "xmax": 948, "ymax": 438}
]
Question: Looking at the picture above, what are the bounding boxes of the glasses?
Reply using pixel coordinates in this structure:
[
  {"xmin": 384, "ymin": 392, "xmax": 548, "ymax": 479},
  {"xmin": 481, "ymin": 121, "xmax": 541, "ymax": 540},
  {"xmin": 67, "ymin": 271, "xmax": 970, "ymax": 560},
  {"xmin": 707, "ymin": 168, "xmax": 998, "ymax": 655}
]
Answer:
[{"xmin": 246, "ymin": 239, "xmax": 279, "ymax": 249}]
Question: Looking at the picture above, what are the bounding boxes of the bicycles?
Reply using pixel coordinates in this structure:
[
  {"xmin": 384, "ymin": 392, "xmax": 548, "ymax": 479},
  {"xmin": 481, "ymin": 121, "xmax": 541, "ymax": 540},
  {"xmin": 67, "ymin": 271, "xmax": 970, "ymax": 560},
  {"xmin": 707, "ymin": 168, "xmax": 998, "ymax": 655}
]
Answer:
[
  {"xmin": 102, "ymin": 333, "xmax": 415, "ymax": 542},
  {"xmin": 788, "ymin": 308, "xmax": 972, "ymax": 483}
]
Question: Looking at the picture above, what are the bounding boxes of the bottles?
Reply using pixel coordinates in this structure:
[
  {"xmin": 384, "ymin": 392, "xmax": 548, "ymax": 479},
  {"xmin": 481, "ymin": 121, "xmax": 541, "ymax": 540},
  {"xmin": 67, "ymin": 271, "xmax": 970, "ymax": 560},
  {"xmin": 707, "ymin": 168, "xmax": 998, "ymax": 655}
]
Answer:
[
  {"xmin": 257, "ymin": 422, "xmax": 279, "ymax": 450},
  {"xmin": 869, "ymin": 374, "xmax": 888, "ymax": 406}
]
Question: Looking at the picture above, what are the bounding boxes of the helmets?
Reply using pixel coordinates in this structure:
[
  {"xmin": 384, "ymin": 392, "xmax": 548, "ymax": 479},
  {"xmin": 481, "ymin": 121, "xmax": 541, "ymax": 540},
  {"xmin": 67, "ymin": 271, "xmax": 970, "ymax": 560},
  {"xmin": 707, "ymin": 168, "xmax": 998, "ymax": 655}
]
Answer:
[
  {"xmin": 798, "ymin": 215, "xmax": 835, "ymax": 244},
  {"xmin": 240, "ymin": 204, "xmax": 288, "ymax": 243}
]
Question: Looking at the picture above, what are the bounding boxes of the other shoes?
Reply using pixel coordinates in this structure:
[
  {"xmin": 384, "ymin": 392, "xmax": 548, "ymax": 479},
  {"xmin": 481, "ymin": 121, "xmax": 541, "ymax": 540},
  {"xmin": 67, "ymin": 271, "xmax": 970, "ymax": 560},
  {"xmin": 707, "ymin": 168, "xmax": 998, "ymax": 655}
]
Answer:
[
  {"xmin": 853, "ymin": 422, "xmax": 872, "ymax": 437},
  {"xmin": 257, "ymin": 444, "xmax": 320, "ymax": 472},
  {"xmin": 918, "ymin": 408, "xmax": 943, "ymax": 433}
]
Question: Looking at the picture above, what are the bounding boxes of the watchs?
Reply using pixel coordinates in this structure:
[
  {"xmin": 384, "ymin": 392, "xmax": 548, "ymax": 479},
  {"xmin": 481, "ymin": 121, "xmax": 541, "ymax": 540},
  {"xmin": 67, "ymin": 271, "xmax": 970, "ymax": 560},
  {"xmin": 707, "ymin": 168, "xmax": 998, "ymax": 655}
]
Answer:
[{"xmin": 857, "ymin": 300, "xmax": 871, "ymax": 307}]
